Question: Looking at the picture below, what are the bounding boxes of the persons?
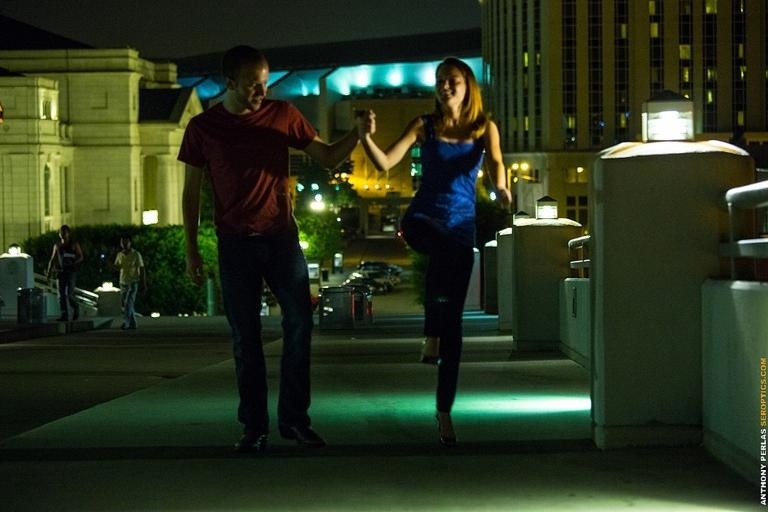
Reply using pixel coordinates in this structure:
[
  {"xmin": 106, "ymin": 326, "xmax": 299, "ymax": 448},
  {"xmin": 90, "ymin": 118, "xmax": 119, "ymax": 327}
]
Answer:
[
  {"xmin": 176, "ymin": 45, "xmax": 376, "ymax": 461},
  {"xmin": 353, "ymin": 55, "xmax": 513, "ymax": 446},
  {"xmin": 113, "ymin": 235, "xmax": 147, "ymax": 330},
  {"xmin": 46, "ymin": 225, "xmax": 84, "ymax": 322}
]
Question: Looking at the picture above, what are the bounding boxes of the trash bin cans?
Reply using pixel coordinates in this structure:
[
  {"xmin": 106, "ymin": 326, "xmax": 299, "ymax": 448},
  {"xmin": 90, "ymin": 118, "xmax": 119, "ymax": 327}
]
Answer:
[
  {"xmin": 333, "ymin": 253, "xmax": 344, "ymax": 274},
  {"xmin": 17, "ymin": 287, "xmax": 45, "ymax": 326},
  {"xmin": 318, "ymin": 286, "xmax": 374, "ymax": 336},
  {"xmin": 307, "ymin": 264, "xmax": 320, "ymax": 285}
]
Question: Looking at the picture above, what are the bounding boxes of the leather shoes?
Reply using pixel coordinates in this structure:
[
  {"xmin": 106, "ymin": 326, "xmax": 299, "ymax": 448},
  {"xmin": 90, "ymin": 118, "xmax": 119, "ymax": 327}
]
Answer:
[
  {"xmin": 422, "ymin": 336, "xmax": 438, "ymax": 366},
  {"xmin": 434, "ymin": 414, "xmax": 457, "ymax": 445},
  {"xmin": 234, "ymin": 432, "xmax": 266, "ymax": 452},
  {"xmin": 279, "ymin": 428, "xmax": 327, "ymax": 458}
]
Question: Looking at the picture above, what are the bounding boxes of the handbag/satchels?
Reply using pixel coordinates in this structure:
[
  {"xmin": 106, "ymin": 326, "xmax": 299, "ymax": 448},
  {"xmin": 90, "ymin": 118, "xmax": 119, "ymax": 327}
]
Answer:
[{"xmin": 475, "ymin": 197, "xmax": 514, "ymax": 240}]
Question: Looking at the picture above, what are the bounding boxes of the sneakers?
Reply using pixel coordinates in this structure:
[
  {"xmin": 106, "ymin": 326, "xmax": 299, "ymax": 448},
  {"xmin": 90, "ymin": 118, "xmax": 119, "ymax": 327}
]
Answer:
[
  {"xmin": 55, "ymin": 317, "xmax": 69, "ymax": 322},
  {"xmin": 72, "ymin": 304, "xmax": 80, "ymax": 320}
]
